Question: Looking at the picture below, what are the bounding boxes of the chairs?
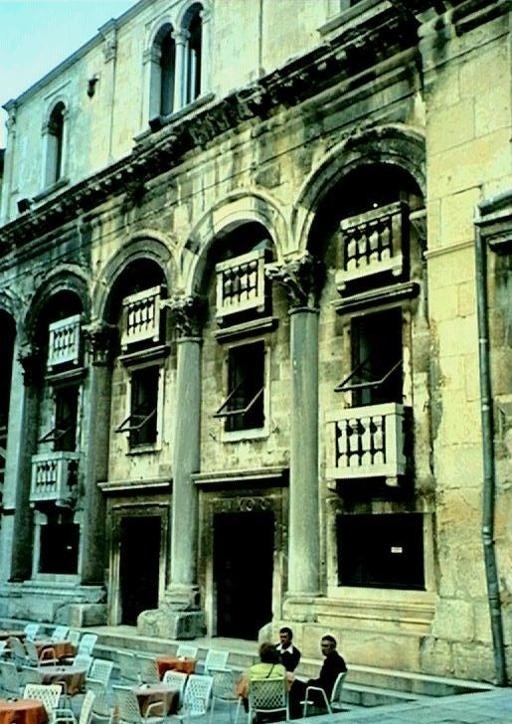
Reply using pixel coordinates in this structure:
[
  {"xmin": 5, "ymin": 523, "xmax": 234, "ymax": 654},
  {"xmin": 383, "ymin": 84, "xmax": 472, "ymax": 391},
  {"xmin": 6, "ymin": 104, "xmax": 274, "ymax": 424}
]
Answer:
[{"xmin": 0, "ymin": 621, "xmax": 348, "ymax": 723}]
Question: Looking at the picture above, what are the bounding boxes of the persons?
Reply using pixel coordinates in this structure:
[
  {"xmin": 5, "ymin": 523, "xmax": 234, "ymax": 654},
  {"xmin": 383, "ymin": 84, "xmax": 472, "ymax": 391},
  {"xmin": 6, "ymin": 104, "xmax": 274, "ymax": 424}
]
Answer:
[
  {"xmin": 290, "ymin": 637, "xmax": 348, "ymax": 720},
  {"xmin": 275, "ymin": 627, "xmax": 300, "ymax": 672},
  {"xmin": 243, "ymin": 643, "xmax": 292, "ymax": 724}
]
